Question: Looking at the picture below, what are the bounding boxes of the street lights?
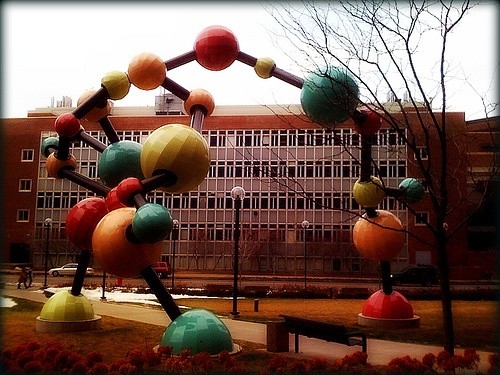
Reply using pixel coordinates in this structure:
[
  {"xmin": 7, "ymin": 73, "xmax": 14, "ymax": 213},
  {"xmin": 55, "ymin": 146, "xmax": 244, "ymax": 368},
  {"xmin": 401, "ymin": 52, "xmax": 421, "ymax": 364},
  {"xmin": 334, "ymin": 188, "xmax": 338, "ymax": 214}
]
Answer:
[
  {"xmin": 170, "ymin": 219, "xmax": 179, "ymax": 290},
  {"xmin": 42, "ymin": 217, "xmax": 50, "ymax": 287},
  {"xmin": 228, "ymin": 185, "xmax": 245, "ymax": 316},
  {"xmin": 302, "ymin": 219, "xmax": 309, "ymax": 291}
]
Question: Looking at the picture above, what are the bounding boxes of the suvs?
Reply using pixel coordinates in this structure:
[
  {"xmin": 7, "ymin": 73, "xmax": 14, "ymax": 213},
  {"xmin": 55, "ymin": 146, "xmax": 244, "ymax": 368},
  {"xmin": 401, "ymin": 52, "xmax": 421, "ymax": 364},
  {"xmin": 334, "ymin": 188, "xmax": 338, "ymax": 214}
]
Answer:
[
  {"xmin": 152, "ymin": 261, "xmax": 172, "ymax": 279},
  {"xmin": 392, "ymin": 263, "xmax": 438, "ymax": 286}
]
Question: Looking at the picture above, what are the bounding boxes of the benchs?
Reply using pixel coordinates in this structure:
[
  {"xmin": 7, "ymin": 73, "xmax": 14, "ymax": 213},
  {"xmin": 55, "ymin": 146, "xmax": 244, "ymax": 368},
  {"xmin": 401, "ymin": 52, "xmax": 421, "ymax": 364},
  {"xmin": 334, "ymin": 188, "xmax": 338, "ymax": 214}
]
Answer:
[
  {"xmin": 243, "ymin": 285, "xmax": 271, "ymax": 297},
  {"xmin": 205, "ymin": 285, "xmax": 234, "ymax": 297},
  {"xmin": 278, "ymin": 313, "xmax": 375, "ymax": 356}
]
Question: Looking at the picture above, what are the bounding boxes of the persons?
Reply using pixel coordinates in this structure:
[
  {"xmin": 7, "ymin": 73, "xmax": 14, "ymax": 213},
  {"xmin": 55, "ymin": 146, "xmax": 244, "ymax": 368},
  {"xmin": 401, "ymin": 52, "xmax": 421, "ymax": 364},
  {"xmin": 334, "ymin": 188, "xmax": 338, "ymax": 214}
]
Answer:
[
  {"xmin": 18, "ymin": 267, "xmax": 30, "ymax": 290},
  {"xmin": 28, "ymin": 265, "xmax": 36, "ymax": 288}
]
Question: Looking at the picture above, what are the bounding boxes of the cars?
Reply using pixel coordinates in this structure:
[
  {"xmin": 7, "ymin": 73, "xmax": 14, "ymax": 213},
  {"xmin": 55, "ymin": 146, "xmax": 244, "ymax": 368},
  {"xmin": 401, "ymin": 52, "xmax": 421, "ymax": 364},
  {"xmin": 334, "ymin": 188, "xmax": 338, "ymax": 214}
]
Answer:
[{"xmin": 49, "ymin": 263, "xmax": 94, "ymax": 277}]
